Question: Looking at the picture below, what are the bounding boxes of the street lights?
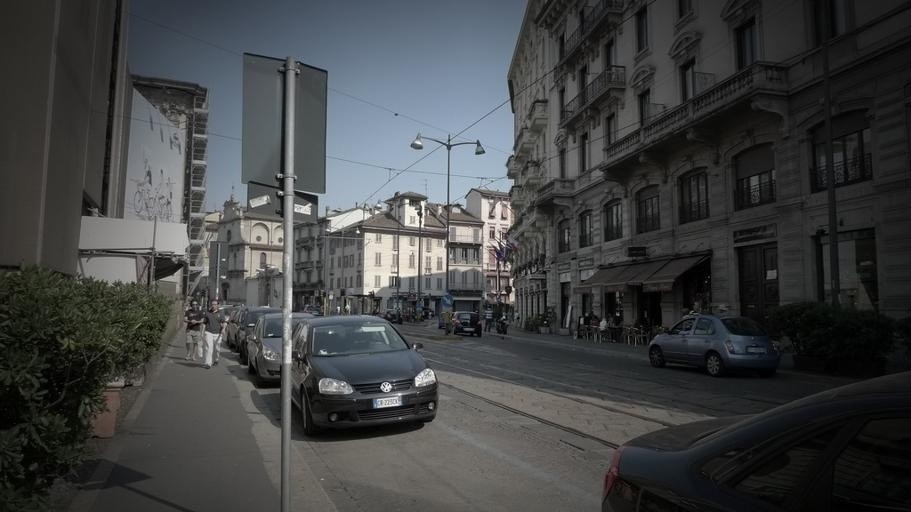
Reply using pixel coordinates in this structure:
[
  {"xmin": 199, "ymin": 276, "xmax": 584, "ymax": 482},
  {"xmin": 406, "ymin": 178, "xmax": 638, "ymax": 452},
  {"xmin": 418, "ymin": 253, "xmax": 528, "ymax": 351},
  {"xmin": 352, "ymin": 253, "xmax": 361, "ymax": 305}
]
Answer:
[
  {"xmin": 414, "ymin": 203, "xmax": 423, "ymax": 304},
  {"xmin": 326, "ymin": 226, "xmax": 360, "ymax": 315},
  {"xmin": 409, "ymin": 132, "xmax": 485, "ymax": 311}
]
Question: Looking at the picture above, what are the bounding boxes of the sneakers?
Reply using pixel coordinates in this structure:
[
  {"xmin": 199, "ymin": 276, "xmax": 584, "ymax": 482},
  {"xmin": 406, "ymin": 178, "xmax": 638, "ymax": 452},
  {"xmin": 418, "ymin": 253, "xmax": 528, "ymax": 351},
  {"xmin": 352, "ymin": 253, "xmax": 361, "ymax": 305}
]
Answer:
[
  {"xmin": 200, "ymin": 364, "xmax": 210, "ymax": 369},
  {"xmin": 185, "ymin": 354, "xmax": 196, "ymax": 360},
  {"xmin": 212, "ymin": 362, "xmax": 217, "ymax": 368}
]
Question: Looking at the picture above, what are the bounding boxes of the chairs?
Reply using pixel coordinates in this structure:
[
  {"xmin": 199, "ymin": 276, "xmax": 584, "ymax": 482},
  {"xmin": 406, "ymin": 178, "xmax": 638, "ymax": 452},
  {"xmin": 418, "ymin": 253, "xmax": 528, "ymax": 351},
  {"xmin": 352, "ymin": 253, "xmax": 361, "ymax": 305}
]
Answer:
[{"xmin": 585, "ymin": 324, "xmax": 650, "ymax": 347}]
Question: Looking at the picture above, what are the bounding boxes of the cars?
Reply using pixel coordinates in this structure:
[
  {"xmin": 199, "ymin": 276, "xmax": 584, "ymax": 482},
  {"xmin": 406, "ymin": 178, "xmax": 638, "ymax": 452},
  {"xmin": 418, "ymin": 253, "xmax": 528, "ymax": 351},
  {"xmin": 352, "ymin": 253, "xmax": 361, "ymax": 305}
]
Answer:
[
  {"xmin": 439, "ymin": 311, "xmax": 482, "ymax": 337},
  {"xmin": 602, "ymin": 371, "xmax": 911, "ymax": 511},
  {"xmin": 222, "ymin": 304, "xmax": 440, "ymax": 437},
  {"xmin": 647, "ymin": 314, "xmax": 782, "ymax": 377}
]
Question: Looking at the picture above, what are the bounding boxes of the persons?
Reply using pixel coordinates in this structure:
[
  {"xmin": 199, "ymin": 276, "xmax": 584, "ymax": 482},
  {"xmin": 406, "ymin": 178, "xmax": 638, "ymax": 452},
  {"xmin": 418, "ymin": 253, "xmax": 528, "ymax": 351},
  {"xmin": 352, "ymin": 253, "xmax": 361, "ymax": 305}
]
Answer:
[
  {"xmin": 514, "ymin": 309, "xmax": 521, "ymax": 328},
  {"xmin": 182, "ymin": 298, "xmax": 229, "ymax": 369},
  {"xmin": 717, "ymin": 304, "xmax": 728, "ymax": 314},
  {"xmin": 498, "ymin": 312, "xmax": 509, "ymax": 327},
  {"xmin": 336, "ymin": 306, "xmax": 340, "ymax": 314},
  {"xmin": 690, "ymin": 302, "xmax": 701, "ymax": 314},
  {"xmin": 584, "ymin": 311, "xmax": 614, "ymax": 343},
  {"xmin": 479, "ymin": 305, "xmax": 494, "ymax": 332},
  {"xmin": 345, "ymin": 303, "xmax": 351, "ymax": 315}
]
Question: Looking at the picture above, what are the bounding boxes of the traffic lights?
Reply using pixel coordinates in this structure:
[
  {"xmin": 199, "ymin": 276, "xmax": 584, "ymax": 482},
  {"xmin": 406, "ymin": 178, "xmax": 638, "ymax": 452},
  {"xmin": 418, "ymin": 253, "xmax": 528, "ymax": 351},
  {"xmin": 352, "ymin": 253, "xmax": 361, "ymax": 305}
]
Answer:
[
  {"xmin": 341, "ymin": 288, "xmax": 346, "ymax": 297},
  {"xmin": 319, "ymin": 290, "xmax": 326, "ymax": 297}
]
